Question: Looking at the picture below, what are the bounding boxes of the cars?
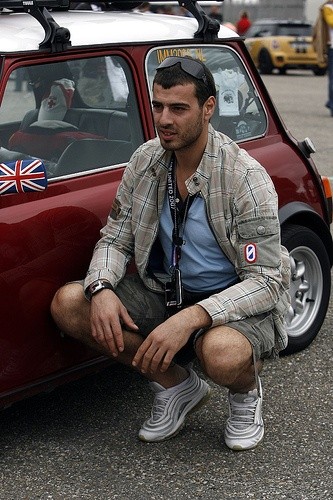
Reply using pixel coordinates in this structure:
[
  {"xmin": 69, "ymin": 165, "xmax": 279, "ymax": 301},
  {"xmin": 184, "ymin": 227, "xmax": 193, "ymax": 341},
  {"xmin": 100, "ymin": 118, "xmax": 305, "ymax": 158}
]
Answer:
[
  {"xmin": 245, "ymin": 17, "xmax": 327, "ymax": 76},
  {"xmin": 0, "ymin": 0, "xmax": 333, "ymax": 411}
]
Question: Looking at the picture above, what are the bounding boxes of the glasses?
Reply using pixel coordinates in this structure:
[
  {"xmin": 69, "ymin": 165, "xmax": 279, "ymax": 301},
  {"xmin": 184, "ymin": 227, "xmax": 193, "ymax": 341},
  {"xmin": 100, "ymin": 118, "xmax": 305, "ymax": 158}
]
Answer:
[{"xmin": 155, "ymin": 56, "xmax": 211, "ymax": 86}]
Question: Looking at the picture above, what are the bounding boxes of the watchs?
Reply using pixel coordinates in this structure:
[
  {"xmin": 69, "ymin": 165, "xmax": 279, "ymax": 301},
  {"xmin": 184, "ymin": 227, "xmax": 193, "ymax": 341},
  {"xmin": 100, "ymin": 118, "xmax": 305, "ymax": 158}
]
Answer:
[{"xmin": 84, "ymin": 281, "xmax": 113, "ymax": 297}]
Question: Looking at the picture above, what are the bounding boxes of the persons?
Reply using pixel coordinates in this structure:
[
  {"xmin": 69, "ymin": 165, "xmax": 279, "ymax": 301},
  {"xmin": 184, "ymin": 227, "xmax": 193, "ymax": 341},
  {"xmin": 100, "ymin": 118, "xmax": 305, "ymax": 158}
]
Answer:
[
  {"xmin": 46, "ymin": 55, "xmax": 293, "ymax": 452},
  {"xmin": 310, "ymin": 0, "xmax": 333, "ymax": 117},
  {"xmin": 205, "ymin": 4, "xmax": 224, "ymax": 24},
  {"xmin": 235, "ymin": 11, "xmax": 251, "ymax": 38},
  {"xmin": 132, "ymin": 1, "xmax": 153, "ymax": 15},
  {"xmin": 13, "ymin": 68, "xmax": 32, "ymax": 93}
]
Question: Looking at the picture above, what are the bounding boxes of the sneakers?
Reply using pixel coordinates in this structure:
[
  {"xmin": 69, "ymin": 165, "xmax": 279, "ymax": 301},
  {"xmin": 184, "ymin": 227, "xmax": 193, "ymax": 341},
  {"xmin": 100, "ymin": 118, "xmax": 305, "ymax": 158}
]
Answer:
[
  {"xmin": 138, "ymin": 369, "xmax": 211, "ymax": 442},
  {"xmin": 224, "ymin": 375, "xmax": 264, "ymax": 451}
]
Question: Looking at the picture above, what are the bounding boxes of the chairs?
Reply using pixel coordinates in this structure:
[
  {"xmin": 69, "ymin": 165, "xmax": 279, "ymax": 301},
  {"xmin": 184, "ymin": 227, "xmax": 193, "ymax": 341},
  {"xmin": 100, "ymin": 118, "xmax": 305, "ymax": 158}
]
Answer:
[{"xmin": 7, "ymin": 108, "xmax": 131, "ymax": 178}]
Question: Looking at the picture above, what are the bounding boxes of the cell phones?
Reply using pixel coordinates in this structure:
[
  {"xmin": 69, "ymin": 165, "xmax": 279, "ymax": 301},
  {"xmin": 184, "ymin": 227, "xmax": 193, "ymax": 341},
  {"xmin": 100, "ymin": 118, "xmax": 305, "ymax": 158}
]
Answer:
[{"xmin": 175, "ymin": 268, "xmax": 182, "ymax": 305}]
